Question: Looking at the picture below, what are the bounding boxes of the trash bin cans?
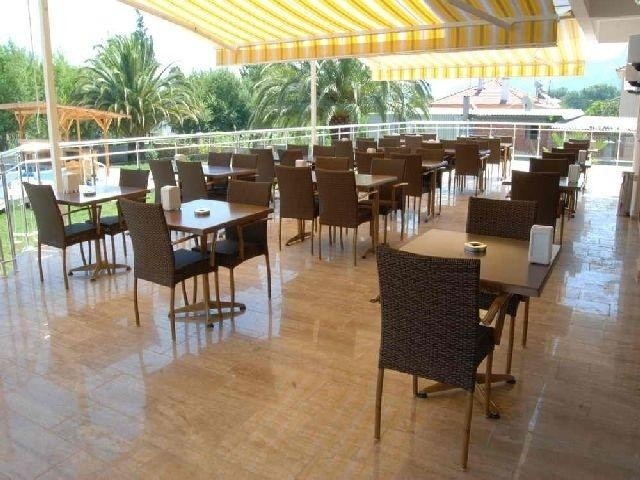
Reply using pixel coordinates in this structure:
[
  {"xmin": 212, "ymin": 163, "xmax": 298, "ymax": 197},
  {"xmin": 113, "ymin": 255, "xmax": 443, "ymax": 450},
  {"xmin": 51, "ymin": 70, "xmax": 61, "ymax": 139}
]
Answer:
[{"xmin": 617, "ymin": 172, "xmax": 634, "ymax": 217}]
